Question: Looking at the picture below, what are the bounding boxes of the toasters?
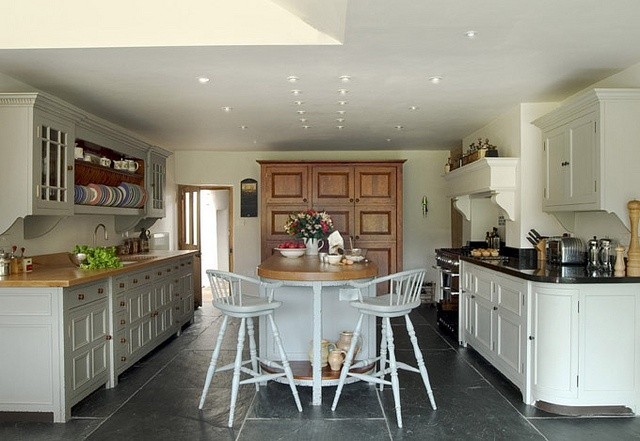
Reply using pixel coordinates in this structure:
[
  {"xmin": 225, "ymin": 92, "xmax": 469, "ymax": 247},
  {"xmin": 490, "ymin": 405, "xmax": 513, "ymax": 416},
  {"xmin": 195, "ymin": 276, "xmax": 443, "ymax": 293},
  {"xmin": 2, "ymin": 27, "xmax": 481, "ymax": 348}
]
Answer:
[{"xmin": 548, "ymin": 233, "xmax": 584, "ymax": 266}]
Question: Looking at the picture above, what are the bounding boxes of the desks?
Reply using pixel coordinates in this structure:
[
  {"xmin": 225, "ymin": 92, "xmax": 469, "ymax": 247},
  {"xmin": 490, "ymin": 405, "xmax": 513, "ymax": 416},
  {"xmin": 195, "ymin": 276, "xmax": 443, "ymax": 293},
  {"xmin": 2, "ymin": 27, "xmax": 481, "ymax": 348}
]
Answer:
[{"xmin": 256, "ymin": 250, "xmax": 377, "ymax": 408}]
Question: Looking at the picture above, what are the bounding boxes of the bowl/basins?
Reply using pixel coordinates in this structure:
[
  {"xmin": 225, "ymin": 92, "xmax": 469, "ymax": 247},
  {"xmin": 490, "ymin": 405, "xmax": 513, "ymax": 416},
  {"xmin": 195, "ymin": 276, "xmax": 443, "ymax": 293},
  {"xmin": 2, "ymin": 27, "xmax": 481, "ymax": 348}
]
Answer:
[
  {"xmin": 344, "ymin": 247, "xmax": 367, "ymax": 262},
  {"xmin": 69, "ymin": 253, "xmax": 93, "ymax": 266},
  {"xmin": 274, "ymin": 247, "xmax": 307, "ymax": 257},
  {"xmin": 319, "ymin": 252, "xmax": 342, "ymax": 265}
]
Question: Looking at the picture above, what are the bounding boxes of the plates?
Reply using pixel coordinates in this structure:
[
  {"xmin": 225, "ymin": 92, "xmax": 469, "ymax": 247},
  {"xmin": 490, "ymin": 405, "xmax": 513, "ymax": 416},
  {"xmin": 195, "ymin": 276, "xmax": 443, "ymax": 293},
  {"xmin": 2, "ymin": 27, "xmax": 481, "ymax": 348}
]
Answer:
[{"xmin": 75, "ymin": 180, "xmax": 148, "ymax": 209}]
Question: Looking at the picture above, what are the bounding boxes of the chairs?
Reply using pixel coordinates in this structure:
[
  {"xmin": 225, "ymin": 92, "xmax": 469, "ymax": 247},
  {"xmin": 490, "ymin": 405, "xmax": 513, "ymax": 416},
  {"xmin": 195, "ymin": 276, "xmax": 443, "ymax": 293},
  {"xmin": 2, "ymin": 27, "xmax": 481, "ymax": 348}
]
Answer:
[
  {"xmin": 197, "ymin": 269, "xmax": 303, "ymax": 427},
  {"xmin": 330, "ymin": 269, "xmax": 438, "ymax": 428}
]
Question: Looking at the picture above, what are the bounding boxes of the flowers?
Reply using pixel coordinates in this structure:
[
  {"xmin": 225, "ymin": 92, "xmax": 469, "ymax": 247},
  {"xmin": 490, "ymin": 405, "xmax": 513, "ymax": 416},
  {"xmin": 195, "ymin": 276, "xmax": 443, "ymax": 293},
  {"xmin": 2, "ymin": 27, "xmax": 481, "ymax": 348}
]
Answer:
[{"xmin": 283, "ymin": 207, "xmax": 333, "ymax": 237}]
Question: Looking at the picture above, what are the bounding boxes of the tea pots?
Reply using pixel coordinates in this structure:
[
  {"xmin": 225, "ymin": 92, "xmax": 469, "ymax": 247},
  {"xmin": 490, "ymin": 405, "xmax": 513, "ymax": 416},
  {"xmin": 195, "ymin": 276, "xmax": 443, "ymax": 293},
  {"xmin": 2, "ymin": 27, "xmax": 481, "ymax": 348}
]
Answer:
[
  {"xmin": 124, "ymin": 159, "xmax": 138, "ymax": 174},
  {"xmin": 113, "ymin": 157, "xmax": 128, "ymax": 171},
  {"xmin": 100, "ymin": 155, "xmax": 112, "ymax": 167}
]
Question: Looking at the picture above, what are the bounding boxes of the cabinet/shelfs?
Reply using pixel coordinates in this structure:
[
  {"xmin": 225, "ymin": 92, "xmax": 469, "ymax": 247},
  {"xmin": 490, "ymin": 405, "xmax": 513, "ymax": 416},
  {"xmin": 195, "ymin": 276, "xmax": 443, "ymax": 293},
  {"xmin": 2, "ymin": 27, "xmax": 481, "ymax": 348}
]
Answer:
[
  {"xmin": 495, "ymin": 270, "xmax": 530, "ymax": 400},
  {"xmin": 457, "ymin": 259, "xmax": 492, "ymax": 367},
  {"xmin": 157, "ymin": 251, "xmax": 196, "ymax": 346},
  {"xmin": 5, "ymin": 94, "xmax": 190, "ymax": 231},
  {"xmin": 64, "ymin": 277, "xmax": 110, "ymax": 401},
  {"xmin": 255, "ymin": 159, "xmax": 404, "ymax": 296},
  {"xmin": 112, "ymin": 260, "xmax": 156, "ymax": 387},
  {"xmin": 530, "ymin": 89, "xmax": 605, "ymax": 211}
]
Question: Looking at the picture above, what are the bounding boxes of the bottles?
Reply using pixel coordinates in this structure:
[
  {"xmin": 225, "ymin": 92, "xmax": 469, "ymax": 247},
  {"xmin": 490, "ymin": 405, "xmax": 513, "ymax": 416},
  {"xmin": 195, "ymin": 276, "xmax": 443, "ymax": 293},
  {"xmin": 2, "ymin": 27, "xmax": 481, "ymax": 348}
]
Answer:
[
  {"xmin": 486, "ymin": 226, "xmax": 500, "ymax": 248},
  {"xmin": 587, "ymin": 237, "xmax": 599, "ymax": 276},
  {"xmin": 600, "ymin": 239, "xmax": 613, "ymax": 274}
]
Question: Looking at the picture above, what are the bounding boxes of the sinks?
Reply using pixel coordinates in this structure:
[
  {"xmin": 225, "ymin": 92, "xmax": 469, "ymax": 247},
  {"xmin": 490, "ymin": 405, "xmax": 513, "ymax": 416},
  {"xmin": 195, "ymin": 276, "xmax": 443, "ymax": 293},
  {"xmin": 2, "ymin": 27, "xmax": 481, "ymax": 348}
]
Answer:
[{"xmin": 119, "ymin": 256, "xmax": 154, "ymax": 262}]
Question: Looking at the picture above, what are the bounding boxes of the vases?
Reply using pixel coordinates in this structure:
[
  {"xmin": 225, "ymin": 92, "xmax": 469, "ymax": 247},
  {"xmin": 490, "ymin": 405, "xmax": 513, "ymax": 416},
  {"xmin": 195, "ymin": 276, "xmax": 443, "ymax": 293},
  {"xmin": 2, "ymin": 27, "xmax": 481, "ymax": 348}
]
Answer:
[{"xmin": 301, "ymin": 237, "xmax": 324, "ymax": 255}]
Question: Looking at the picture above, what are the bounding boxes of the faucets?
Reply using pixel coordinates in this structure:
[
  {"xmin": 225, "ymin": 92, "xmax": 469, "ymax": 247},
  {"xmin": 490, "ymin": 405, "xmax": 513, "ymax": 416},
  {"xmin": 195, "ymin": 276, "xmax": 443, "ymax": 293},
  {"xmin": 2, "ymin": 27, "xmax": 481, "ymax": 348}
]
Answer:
[{"xmin": 93, "ymin": 223, "xmax": 109, "ymax": 247}]
